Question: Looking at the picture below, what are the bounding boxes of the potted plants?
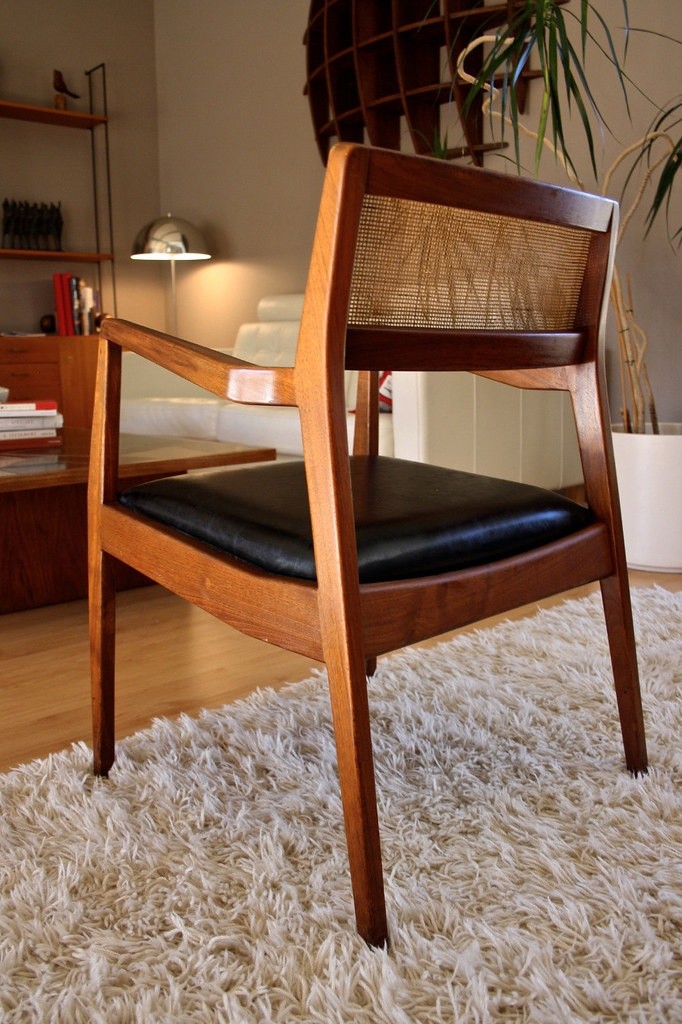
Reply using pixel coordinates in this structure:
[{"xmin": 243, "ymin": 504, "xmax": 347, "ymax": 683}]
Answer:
[{"xmin": 413, "ymin": 1, "xmax": 682, "ymax": 573}]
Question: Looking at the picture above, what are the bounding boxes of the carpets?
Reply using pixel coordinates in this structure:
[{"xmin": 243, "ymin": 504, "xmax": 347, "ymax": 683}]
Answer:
[{"xmin": 0, "ymin": 582, "xmax": 682, "ymax": 1024}]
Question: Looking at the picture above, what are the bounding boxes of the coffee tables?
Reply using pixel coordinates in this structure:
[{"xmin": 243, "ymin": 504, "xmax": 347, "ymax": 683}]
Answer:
[{"xmin": 0, "ymin": 429, "xmax": 278, "ymax": 615}]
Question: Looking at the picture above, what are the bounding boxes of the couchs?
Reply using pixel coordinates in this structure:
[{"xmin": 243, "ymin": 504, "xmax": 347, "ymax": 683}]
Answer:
[{"xmin": 121, "ymin": 292, "xmax": 584, "ymax": 500}]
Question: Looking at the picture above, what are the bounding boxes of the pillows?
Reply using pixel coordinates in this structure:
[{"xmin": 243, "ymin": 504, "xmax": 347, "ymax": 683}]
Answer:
[{"xmin": 349, "ymin": 372, "xmax": 392, "ymax": 414}]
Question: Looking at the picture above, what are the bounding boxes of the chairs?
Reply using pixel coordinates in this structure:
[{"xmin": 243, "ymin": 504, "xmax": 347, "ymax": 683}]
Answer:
[{"xmin": 86, "ymin": 141, "xmax": 648, "ymax": 950}]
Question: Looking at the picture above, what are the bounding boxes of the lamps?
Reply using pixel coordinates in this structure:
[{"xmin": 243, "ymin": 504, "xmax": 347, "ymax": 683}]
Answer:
[{"xmin": 129, "ymin": 212, "xmax": 212, "ymax": 337}]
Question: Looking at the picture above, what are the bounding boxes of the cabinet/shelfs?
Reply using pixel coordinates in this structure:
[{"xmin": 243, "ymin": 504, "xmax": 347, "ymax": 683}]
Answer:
[{"xmin": 0, "ymin": 100, "xmax": 118, "ymax": 427}]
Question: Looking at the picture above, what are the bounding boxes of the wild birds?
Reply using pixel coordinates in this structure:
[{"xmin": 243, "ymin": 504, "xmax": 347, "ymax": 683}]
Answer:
[{"xmin": 52, "ymin": 68, "xmax": 81, "ymax": 100}]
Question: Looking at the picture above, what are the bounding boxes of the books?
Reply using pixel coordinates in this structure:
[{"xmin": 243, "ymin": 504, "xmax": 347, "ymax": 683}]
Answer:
[
  {"xmin": 0, "ymin": 400, "xmax": 64, "ymax": 451},
  {"xmin": 53, "ymin": 273, "xmax": 99, "ymax": 335},
  {"xmin": 1, "ymin": 330, "xmax": 46, "ymax": 337}
]
